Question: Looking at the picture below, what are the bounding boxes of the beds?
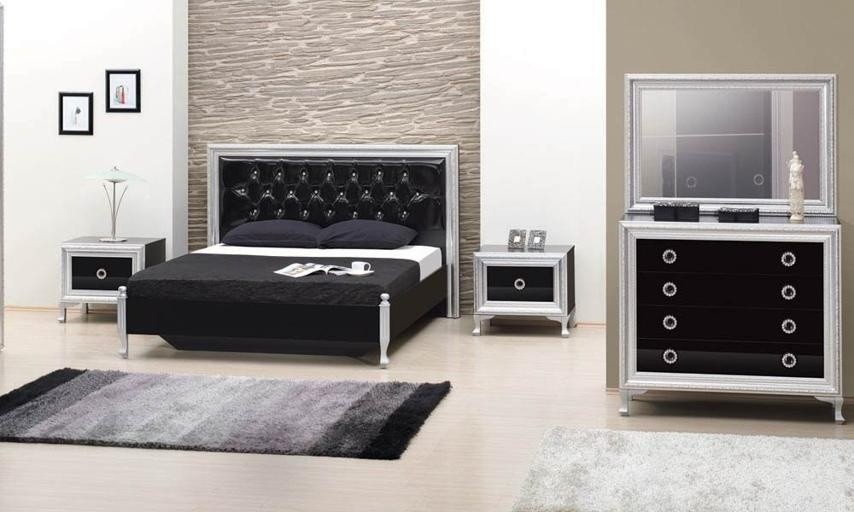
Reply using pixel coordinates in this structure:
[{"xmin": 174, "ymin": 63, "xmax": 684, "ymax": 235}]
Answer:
[{"xmin": 116, "ymin": 145, "xmax": 462, "ymax": 366}]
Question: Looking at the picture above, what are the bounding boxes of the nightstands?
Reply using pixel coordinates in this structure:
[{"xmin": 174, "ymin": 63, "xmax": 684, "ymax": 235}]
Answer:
[
  {"xmin": 471, "ymin": 244, "xmax": 577, "ymax": 334},
  {"xmin": 59, "ymin": 236, "xmax": 166, "ymax": 323}
]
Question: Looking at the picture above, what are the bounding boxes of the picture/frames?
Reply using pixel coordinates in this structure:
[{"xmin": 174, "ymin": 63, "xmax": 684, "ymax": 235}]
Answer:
[
  {"xmin": 104, "ymin": 68, "xmax": 141, "ymax": 113},
  {"xmin": 57, "ymin": 90, "xmax": 95, "ymax": 136},
  {"xmin": 509, "ymin": 228, "xmax": 546, "ymax": 250}
]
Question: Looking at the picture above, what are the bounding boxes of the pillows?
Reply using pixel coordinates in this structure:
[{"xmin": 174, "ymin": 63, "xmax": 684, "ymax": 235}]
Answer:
[{"xmin": 224, "ymin": 219, "xmax": 418, "ymax": 247}]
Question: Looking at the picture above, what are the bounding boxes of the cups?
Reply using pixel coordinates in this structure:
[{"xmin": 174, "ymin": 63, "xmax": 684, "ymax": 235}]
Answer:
[{"xmin": 351, "ymin": 261, "xmax": 369, "ymax": 273}]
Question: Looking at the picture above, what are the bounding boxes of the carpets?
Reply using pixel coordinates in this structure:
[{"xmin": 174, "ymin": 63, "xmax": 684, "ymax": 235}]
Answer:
[
  {"xmin": 0, "ymin": 365, "xmax": 453, "ymax": 464},
  {"xmin": 521, "ymin": 422, "xmax": 854, "ymax": 512}
]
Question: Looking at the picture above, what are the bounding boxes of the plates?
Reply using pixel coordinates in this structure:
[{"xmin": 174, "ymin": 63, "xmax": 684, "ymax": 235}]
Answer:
[{"xmin": 346, "ymin": 271, "xmax": 375, "ymax": 278}]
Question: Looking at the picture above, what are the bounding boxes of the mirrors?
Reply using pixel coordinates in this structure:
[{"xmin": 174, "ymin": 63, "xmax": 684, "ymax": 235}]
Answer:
[{"xmin": 622, "ymin": 72, "xmax": 839, "ymax": 219}]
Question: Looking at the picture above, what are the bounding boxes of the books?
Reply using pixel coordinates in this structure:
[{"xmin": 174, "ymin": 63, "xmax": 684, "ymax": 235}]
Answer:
[{"xmin": 274, "ymin": 262, "xmax": 351, "ymax": 277}]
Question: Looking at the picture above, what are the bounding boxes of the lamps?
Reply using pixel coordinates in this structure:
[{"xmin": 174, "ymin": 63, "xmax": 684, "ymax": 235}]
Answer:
[{"xmin": 100, "ymin": 168, "xmax": 129, "ymax": 243}]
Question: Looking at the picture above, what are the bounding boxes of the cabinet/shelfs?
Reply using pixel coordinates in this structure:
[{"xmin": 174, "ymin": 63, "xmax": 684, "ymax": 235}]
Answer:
[{"xmin": 614, "ymin": 220, "xmax": 845, "ymax": 426}]
